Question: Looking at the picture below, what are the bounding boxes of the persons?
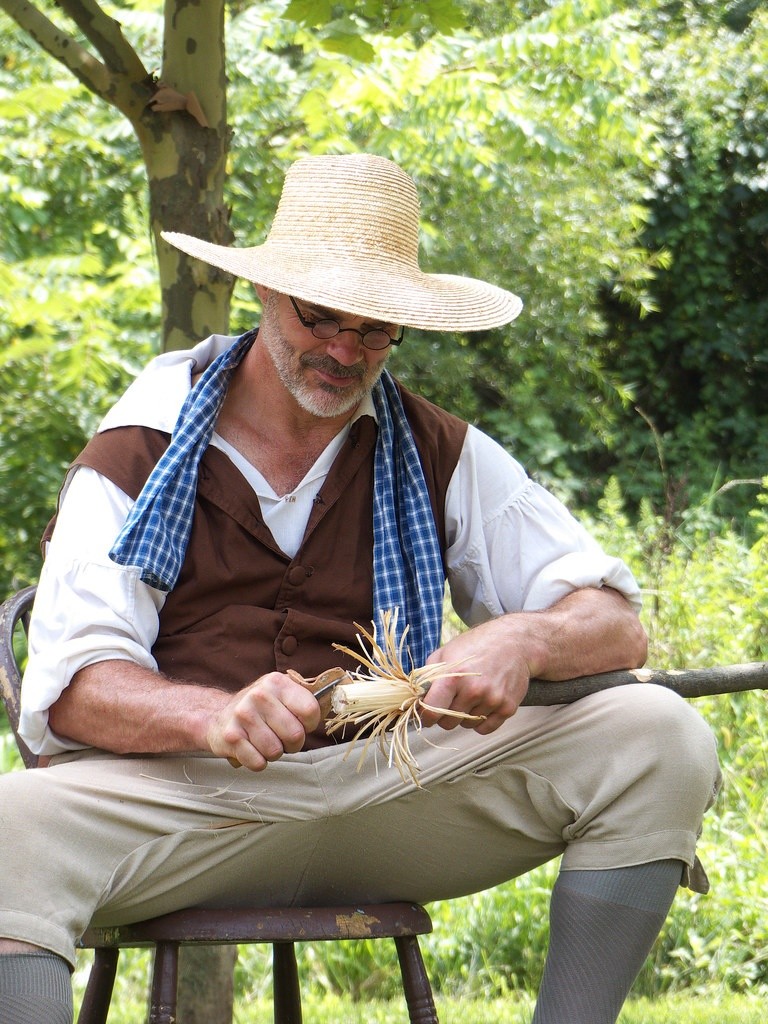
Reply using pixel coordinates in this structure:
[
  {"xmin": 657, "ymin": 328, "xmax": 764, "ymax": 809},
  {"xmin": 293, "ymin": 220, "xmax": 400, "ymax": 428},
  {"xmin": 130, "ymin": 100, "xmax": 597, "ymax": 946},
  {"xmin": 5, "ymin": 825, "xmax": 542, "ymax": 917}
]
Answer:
[{"xmin": 0, "ymin": 154, "xmax": 721, "ymax": 1024}]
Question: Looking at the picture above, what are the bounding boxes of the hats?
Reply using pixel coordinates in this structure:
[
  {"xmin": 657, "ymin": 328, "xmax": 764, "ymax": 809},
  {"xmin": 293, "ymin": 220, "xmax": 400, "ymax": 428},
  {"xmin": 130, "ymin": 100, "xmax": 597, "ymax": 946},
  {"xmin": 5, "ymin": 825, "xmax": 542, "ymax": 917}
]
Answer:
[{"xmin": 159, "ymin": 153, "xmax": 523, "ymax": 332}]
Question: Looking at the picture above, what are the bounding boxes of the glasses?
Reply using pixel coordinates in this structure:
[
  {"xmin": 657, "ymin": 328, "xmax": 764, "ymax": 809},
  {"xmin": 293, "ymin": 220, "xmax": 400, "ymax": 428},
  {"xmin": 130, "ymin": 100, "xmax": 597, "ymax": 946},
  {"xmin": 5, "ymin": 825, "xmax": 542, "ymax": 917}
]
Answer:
[{"xmin": 288, "ymin": 296, "xmax": 404, "ymax": 351}]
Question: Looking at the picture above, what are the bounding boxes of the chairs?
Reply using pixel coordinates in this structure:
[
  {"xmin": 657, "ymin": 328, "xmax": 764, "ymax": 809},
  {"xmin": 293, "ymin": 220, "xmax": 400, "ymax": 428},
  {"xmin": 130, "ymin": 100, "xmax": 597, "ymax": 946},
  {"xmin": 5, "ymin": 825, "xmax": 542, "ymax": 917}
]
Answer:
[{"xmin": 1, "ymin": 585, "xmax": 438, "ymax": 1024}]
